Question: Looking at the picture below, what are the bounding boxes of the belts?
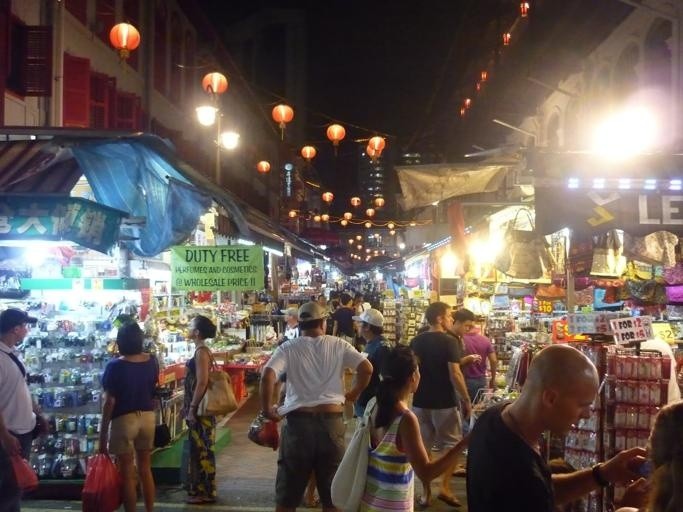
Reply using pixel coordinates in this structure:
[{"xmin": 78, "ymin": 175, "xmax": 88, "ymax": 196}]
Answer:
[{"xmin": 288, "ymin": 410, "xmax": 343, "ymax": 420}]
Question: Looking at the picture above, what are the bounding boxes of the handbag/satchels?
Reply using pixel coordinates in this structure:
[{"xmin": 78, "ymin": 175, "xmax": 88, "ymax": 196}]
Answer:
[
  {"xmin": 189, "ymin": 369, "xmax": 241, "ymax": 420},
  {"xmin": 152, "ymin": 421, "xmax": 171, "ymax": 448},
  {"xmin": 30, "ymin": 411, "xmax": 42, "ymax": 440},
  {"xmin": 248, "ymin": 409, "xmax": 266, "ymax": 446},
  {"xmin": 328, "ymin": 394, "xmax": 385, "ymax": 512}
]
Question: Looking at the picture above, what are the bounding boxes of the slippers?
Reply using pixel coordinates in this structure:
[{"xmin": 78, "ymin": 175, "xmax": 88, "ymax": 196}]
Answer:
[
  {"xmin": 415, "ymin": 491, "xmax": 432, "ymax": 508},
  {"xmin": 436, "ymin": 488, "xmax": 463, "ymax": 508},
  {"xmin": 184, "ymin": 493, "xmax": 218, "ymax": 504}
]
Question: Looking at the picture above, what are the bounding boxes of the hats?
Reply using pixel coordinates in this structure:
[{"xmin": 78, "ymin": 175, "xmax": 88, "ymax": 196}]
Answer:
[
  {"xmin": 295, "ymin": 300, "xmax": 330, "ymax": 324},
  {"xmin": 350, "ymin": 307, "xmax": 385, "ymax": 327},
  {"xmin": 279, "ymin": 306, "xmax": 299, "ymax": 317},
  {"xmin": 0, "ymin": 306, "xmax": 40, "ymax": 330}
]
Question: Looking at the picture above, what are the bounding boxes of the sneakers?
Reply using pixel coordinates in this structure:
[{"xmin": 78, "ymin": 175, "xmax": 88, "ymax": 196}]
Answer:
[{"xmin": 452, "ymin": 462, "xmax": 466, "ymax": 476}]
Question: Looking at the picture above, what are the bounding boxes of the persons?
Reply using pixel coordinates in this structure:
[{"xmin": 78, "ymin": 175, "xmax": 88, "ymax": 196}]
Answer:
[
  {"xmin": 462, "ymin": 321, "xmax": 496, "ymax": 453},
  {"xmin": 360, "ymin": 346, "xmax": 469, "ymax": 512},
  {"xmin": 257, "ymin": 300, "xmax": 374, "ymax": 512},
  {"xmin": 176, "ymin": 315, "xmax": 218, "ymax": 502},
  {"xmin": 275, "ymin": 307, "xmax": 299, "ymax": 409},
  {"xmin": 410, "ymin": 303, "xmax": 471, "ymax": 505},
  {"xmin": 0, "ymin": 309, "xmax": 50, "ymax": 510},
  {"xmin": 449, "ymin": 308, "xmax": 483, "ymax": 476},
  {"xmin": 465, "ymin": 343, "xmax": 652, "ymax": 512},
  {"xmin": 353, "ymin": 308, "xmax": 391, "ymax": 421},
  {"xmin": 614, "ymin": 347, "xmax": 682, "ymax": 511},
  {"xmin": 96, "ymin": 318, "xmax": 160, "ymax": 512},
  {"xmin": 319, "ymin": 289, "xmax": 379, "ymax": 373}
]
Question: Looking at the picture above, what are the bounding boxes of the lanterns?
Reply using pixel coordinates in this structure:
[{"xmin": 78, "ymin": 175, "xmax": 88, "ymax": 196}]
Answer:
[
  {"xmin": 366, "ymin": 136, "xmax": 386, "ymax": 153},
  {"xmin": 458, "ymin": 0, "xmax": 531, "ymax": 117},
  {"xmin": 301, "ymin": 146, "xmax": 316, "ymax": 162},
  {"xmin": 108, "ymin": 20, "xmax": 141, "ymax": 61},
  {"xmin": 202, "ymin": 70, "xmax": 227, "ymax": 100},
  {"xmin": 280, "ymin": 191, "xmax": 416, "ymax": 263},
  {"xmin": 256, "ymin": 159, "xmax": 271, "ymax": 177},
  {"xmin": 364, "ymin": 144, "xmax": 384, "ymax": 162},
  {"xmin": 273, "ymin": 103, "xmax": 294, "ymax": 129},
  {"xmin": 326, "ymin": 124, "xmax": 346, "ymax": 145}
]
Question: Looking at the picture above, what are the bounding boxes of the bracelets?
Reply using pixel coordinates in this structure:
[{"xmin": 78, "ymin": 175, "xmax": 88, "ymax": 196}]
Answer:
[{"xmin": 590, "ymin": 461, "xmax": 612, "ymax": 489}]
